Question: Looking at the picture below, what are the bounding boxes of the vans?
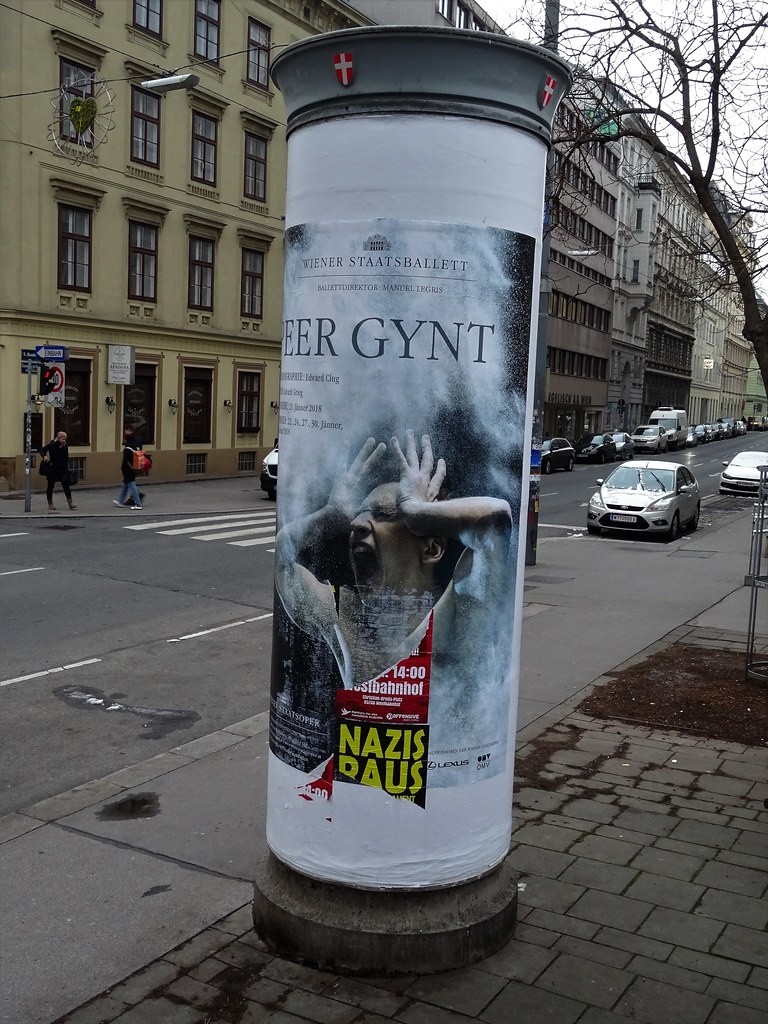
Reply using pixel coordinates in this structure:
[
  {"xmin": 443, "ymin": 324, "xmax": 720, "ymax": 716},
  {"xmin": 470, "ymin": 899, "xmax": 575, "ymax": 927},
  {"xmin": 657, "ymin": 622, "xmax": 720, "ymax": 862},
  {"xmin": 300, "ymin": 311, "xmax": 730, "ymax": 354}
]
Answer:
[{"xmin": 648, "ymin": 407, "xmax": 688, "ymax": 452}]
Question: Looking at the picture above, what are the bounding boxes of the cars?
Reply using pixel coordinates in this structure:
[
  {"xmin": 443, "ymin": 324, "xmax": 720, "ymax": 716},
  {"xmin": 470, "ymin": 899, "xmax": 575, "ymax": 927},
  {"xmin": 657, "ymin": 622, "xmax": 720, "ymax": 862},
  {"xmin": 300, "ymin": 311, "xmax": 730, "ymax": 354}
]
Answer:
[
  {"xmin": 708, "ymin": 423, "xmax": 725, "ymax": 441},
  {"xmin": 717, "ymin": 423, "xmax": 732, "ymax": 440},
  {"xmin": 586, "ymin": 459, "xmax": 701, "ymax": 543},
  {"xmin": 260, "ymin": 442, "xmax": 278, "ymax": 499},
  {"xmin": 541, "ymin": 437, "xmax": 575, "ymax": 474},
  {"xmin": 736, "ymin": 422, "xmax": 742, "ymax": 436},
  {"xmin": 573, "ymin": 432, "xmax": 616, "ymax": 465},
  {"xmin": 703, "ymin": 424, "xmax": 715, "ymax": 441},
  {"xmin": 686, "ymin": 426, "xmax": 698, "ymax": 447},
  {"xmin": 718, "ymin": 450, "xmax": 768, "ymax": 497},
  {"xmin": 604, "ymin": 432, "xmax": 636, "ymax": 461},
  {"xmin": 738, "ymin": 421, "xmax": 747, "ymax": 436},
  {"xmin": 689, "ymin": 424, "xmax": 708, "ymax": 444}
]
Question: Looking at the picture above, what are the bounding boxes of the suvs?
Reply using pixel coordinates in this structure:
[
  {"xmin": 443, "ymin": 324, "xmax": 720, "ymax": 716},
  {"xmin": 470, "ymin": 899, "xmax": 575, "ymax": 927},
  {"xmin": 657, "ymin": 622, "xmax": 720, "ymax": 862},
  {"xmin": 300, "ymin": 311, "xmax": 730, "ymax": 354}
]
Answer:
[
  {"xmin": 747, "ymin": 416, "xmax": 765, "ymax": 432},
  {"xmin": 716, "ymin": 418, "xmax": 737, "ymax": 439},
  {"xmin": 631, "ymin": 424, "xmax": 669, "ymax": 454}
]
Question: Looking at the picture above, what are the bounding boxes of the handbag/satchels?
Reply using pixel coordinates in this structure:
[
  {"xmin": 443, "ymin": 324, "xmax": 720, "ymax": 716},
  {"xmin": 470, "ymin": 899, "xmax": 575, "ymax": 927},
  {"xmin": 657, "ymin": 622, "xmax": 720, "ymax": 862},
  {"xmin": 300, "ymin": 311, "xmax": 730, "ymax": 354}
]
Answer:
[{"xmin": 38, "ymin": 459, "xmax": 50, "ymax": 476}]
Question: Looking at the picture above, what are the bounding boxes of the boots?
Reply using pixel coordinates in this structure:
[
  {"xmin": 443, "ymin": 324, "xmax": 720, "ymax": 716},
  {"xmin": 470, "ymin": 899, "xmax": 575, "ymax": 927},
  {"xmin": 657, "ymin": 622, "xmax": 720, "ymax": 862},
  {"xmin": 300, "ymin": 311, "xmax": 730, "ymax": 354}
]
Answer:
[
  {"xmin": 48, "ymin": 500, "xmax": 56, "ymax": 510},
  {"xmin": 67, "ymin": 497, "xmax": 77, "ymax": 509}
]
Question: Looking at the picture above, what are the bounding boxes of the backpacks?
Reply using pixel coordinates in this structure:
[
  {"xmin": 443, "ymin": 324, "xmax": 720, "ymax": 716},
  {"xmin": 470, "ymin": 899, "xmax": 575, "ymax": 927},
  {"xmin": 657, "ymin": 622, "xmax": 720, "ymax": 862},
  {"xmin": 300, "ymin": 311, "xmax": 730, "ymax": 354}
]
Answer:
[{"xmin": 124, "ymin": 447, "xmax": 148, "ymax": 471}]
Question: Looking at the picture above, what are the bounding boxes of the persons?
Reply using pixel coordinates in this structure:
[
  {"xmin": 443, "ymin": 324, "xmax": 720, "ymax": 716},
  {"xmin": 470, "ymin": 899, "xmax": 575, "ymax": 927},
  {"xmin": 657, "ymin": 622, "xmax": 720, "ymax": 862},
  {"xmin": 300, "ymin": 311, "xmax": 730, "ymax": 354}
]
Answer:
[
  {"xmin": 122, "ymin": 425, "xmax": 147, "ymax": 506},
  {"xmin": 110, "ymin": 434, "xmax": 144, "ymax": 511},
  {"xmin": 39, "ymin": 431, "xmax": 78, "ymax": 511},
  {"xmin": 271, "ymin": 428, "xmax": 519, "ymax": 730}
]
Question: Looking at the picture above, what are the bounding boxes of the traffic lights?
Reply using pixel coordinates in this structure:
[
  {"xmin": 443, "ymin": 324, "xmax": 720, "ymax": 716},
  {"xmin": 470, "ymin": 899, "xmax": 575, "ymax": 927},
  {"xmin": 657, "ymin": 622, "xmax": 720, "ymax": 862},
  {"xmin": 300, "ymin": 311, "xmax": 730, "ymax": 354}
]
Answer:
[{"xmin": 40, "ymin": 366, "xmax": 56, "ymax": 396}]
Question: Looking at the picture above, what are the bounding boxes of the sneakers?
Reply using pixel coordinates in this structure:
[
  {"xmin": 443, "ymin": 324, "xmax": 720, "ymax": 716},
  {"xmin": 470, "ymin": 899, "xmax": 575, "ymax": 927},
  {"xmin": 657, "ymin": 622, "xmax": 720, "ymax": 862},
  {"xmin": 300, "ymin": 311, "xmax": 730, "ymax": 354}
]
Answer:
[
  {"xmin": 131, "ymin": 506, "xmax": 143, "ymax": 510},
  {"xmin": 112, "ymin": 500, "xmax": 125, "ymax": 508}
]
